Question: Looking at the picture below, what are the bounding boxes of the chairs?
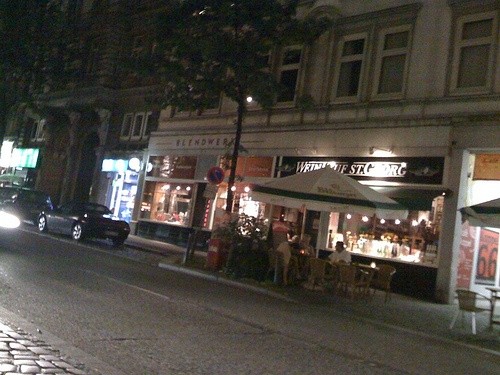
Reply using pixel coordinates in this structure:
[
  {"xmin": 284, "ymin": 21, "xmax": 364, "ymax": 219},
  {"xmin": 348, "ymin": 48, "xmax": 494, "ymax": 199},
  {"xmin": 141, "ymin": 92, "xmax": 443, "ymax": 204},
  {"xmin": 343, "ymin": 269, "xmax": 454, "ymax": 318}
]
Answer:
[
  {"xmin": 308, "ymin": 256, "xmax": 340, "ymax": 295},
  {"xmin": 448, "ymin": 287, "xmax": 494, "ymax": 336},
  {"xmin": 361, "ymin": 262, "xmax": 398, "ymax": 305},
  {"xmin": 331, "ymin": 260, "xmax": 369, "ymax": 300},
  {"xmin": 264, "ymin": 248, "xmax": 295, "ymax": 288}
]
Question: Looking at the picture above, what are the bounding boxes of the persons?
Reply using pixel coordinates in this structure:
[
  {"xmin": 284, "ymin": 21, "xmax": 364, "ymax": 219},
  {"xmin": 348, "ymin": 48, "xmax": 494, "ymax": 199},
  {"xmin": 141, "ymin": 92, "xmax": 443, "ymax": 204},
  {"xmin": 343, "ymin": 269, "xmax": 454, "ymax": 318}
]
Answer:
[
  {"xmin": 153, "ymin": 205, "xmax": 191, "ymax": 223},
  {"xmin": 277, "ymin": 240, "xmax": 301, "ymax": 286},
  {"xmin": 326, "ymin": 241, "xmax": 353, "ymax": 267},
  {"xmin": 301, "ymin": 233, "xmax": 316, "ymax": 257}
]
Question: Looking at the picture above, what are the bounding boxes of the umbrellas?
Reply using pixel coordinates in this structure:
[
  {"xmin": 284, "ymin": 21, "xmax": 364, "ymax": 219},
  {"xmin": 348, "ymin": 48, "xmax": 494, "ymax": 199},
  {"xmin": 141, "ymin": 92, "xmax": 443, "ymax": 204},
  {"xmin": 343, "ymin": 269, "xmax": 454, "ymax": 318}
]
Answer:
[
  {"xmin": 249, "ymin": 166, "xmax": 409, "ymax": 240},
  {"xmin": 456, "ymin": 197, "xmax": 500, "ymax": 230}
]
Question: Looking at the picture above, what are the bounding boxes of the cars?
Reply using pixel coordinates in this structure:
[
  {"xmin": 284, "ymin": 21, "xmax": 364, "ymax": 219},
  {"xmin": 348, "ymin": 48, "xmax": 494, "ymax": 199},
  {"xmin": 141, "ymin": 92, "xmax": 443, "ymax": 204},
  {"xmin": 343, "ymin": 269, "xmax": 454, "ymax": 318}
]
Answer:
[
  {"xmin": 0, "ymin": 183, "xmax": 53, "ymax": 226},
  {"xmin": 38, "ymin": 202, "xmax": 130, "ymax": 247}
]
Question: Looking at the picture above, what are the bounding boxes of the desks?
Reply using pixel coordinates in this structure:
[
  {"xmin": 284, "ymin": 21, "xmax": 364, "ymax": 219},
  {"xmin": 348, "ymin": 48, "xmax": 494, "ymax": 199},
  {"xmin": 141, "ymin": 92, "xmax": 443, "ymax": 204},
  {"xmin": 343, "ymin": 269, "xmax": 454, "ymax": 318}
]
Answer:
[
  {"xmin": 486, "ymin": 284, "xmax": 500, "ymax": 331},
  {"xmin": 293, "ymin": 251, "xmax": 310, "ymax": 286},
  {"xmin": 344, "ymin": 258, "xmax": 381, "ymax": 297}
]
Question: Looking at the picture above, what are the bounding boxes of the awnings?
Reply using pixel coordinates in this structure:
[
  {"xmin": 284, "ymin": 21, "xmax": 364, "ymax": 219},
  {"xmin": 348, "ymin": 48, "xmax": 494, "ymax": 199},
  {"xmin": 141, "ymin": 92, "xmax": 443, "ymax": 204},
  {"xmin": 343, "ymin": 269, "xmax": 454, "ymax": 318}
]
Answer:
[{"xmin": 377, "ymin": 188, "xmax": 453, "ymax": 212}]
